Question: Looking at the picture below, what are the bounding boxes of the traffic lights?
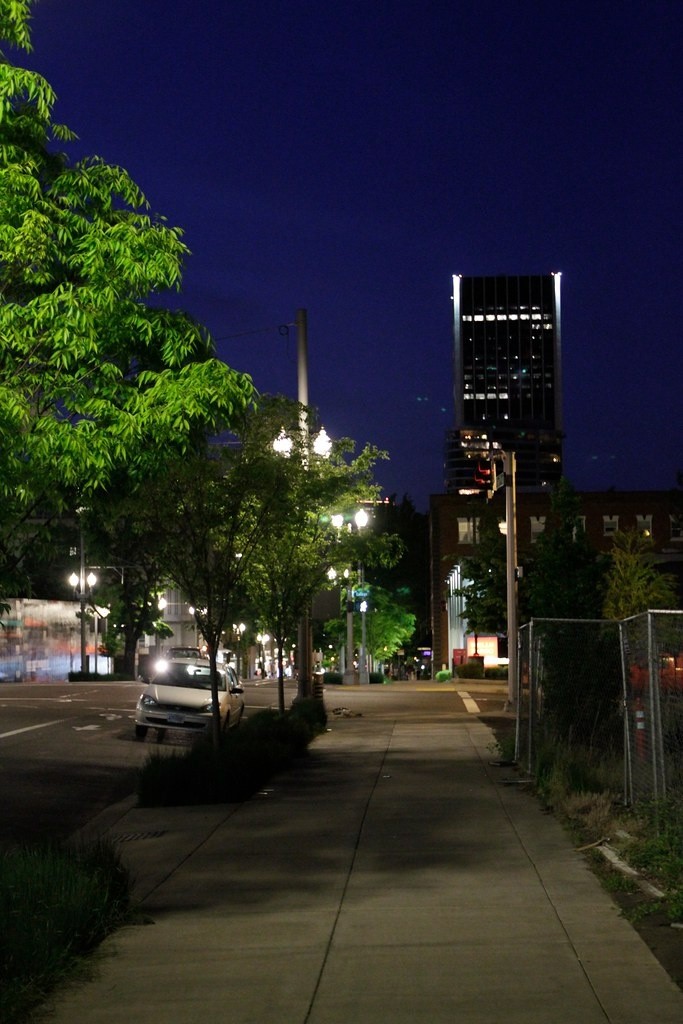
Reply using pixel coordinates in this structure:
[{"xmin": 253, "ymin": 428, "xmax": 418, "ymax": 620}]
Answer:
[
  {"xmin": 341, "ymin": 599, "xmax": 353, "ymax": 613},
  {"xmin": 473, "ymin": 456, "xmax": 493, "ymax": 490},
  {"xmin": 354, "ymin": 600, "xmax": 368, "ymax": 613}
]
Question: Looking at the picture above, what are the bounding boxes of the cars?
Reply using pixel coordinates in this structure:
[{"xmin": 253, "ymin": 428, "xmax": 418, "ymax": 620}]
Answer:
[{"xmin": 133, "ymin": 646, "xmax": 244, "ymax": 741}]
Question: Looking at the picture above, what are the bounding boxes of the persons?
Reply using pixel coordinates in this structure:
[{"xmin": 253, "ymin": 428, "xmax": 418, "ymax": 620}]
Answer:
[
  {"xmin": 407, "ymin": 665, "xmax": 413, "ymax": 680},
  {"xmin": 417, "ymin": 668, "xmax": 421, "ymax": 680}
]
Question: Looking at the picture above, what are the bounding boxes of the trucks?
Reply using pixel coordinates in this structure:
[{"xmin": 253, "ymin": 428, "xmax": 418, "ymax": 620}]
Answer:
[{"xmin": 627, "ymin": 650, "xmax": 683, "ymax": 709}]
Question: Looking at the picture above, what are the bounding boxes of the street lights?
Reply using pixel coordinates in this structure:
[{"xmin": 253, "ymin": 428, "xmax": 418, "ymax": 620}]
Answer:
[
  {"xmin": 233, "ymin": 623, "xmax": 247, "ymax": 677},
  {"xmin": 327, "ymin": 565, "xmax": 349, "ymax": 674},
  {"xmin": 188, "ymin": 607, "xmax": 209, "ymax": 647},
  {"xmin": 257, "ymin": 633, "xmax": 271, "ymax": 679},
  {"xmin": 273, "ymin": 426, "xmax": 333, "ymax": 704},
  {"xmin": 329, "ymin": 508, "xmax": 370, "ymax": 685},
  {"xmin": 155, "ymin": 597, "xmax": 169, "ymax": 647},
  {"xmin": 68, "ymin": 507, "xmax": 97, "ymax": 673}
]
